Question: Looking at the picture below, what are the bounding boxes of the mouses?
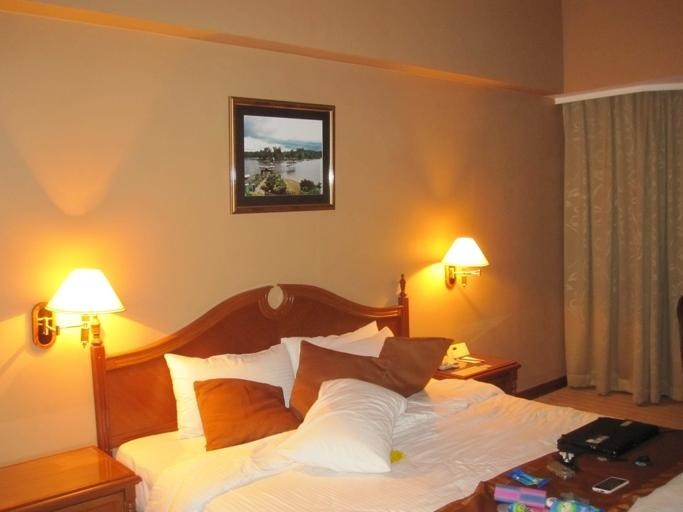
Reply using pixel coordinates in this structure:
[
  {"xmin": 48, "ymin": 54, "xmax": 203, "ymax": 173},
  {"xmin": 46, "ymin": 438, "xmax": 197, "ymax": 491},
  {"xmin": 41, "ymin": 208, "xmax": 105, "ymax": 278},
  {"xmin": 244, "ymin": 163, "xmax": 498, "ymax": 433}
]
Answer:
[{"xmin": 634, "ymin": 456, "xmax": 652, "ymax": 466}]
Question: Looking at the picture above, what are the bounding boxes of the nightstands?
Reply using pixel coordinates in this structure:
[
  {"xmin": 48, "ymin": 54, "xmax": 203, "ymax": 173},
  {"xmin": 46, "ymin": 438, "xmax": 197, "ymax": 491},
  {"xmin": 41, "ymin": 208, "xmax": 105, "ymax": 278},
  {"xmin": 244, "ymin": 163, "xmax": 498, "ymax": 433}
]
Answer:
[
  {"xmin": 432, "ymin": 358, "xmax": 520, "ymax": 396},
  {"xmin": 1, "ymin": 446, "xmax": 144, "ymax": 512}
]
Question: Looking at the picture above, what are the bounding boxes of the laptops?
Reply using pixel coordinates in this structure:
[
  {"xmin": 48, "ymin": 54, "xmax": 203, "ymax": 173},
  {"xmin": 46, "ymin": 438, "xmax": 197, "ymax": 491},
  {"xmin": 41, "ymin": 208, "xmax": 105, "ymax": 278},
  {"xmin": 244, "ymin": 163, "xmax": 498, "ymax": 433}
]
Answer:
[{"xmin": 556, "ymin": 417, "xmax": 659, "ymax": 458}]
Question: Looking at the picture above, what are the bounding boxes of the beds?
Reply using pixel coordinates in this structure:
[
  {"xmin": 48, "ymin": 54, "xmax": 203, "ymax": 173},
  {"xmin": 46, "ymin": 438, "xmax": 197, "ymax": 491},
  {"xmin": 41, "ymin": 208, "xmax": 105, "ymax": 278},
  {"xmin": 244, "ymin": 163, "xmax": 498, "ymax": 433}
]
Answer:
[{"xmin": 88, "ymin": 276, "xmax": 682, "ymax": 510}]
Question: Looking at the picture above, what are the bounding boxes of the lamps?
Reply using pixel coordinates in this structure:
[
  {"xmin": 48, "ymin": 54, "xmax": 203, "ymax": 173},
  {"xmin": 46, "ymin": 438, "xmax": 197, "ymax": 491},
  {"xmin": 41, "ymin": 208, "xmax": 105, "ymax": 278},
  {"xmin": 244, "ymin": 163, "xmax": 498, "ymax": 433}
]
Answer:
[
  {"xmin": 31, "ymin": 266, "xmax": 125, "ymax": 351},
  {"xmin": 445, "ymin": 237, "xmax": 490, "ymax": 291}
]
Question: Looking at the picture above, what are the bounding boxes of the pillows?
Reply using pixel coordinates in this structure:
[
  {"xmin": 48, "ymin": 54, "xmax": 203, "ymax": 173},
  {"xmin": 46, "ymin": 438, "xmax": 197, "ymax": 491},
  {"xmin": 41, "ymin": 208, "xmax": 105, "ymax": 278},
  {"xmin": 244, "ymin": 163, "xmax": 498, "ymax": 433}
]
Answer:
[
  {"xmin": 375, "ymin": 338, "xmax": 456, "ymax": 393},
  {"xmin": 292, "ymin": 326, "xmax": 396, "ymax": 371},
  {"xmin": 162, "ymin": 344, "xmax": 294, "ymax": 440},
  {"xmin": 192, "ymin": 378, "xmax": 300, "ymax": 451},
  {"xmin": 283, "ymin": 341, "xmax": 417, "ymax": 430},
  {"xmin": 273, "ymin": 378, "xmax": 408, "ymax": 474},
  {"xmin": 279, "ymin": 320, "xmax": 384, "ymax": 370}
]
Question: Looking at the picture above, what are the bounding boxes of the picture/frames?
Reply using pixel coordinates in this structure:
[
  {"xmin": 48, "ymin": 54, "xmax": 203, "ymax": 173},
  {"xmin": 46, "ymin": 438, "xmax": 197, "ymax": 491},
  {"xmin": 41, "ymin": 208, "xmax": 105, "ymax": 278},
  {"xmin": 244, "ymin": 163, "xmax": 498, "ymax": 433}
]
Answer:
[{"xmin": 227, "ymin": 96, "xmax": 336, "ymax": 214}]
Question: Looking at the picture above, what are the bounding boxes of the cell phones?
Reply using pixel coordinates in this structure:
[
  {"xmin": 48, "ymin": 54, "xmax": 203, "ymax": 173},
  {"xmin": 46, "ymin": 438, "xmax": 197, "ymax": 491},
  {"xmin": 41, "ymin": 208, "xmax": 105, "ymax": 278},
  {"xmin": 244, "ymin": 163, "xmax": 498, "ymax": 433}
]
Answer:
[{"xmin": 592, "ymin": 475, "xmax": 630, "ymax": 496}]
